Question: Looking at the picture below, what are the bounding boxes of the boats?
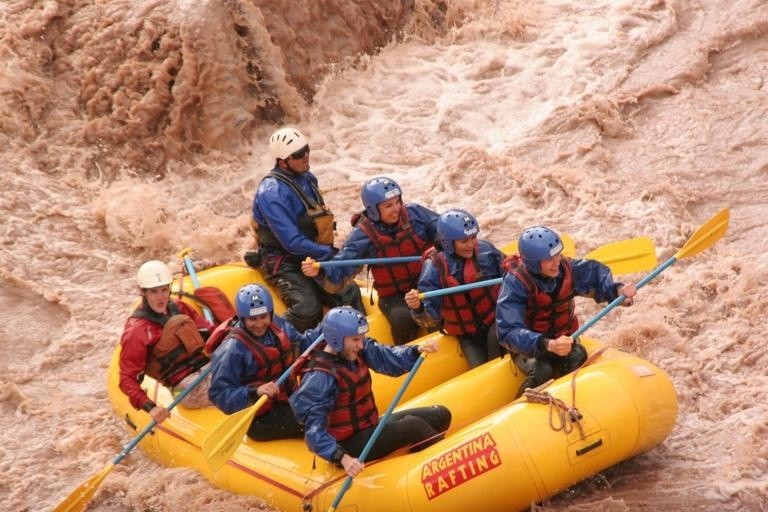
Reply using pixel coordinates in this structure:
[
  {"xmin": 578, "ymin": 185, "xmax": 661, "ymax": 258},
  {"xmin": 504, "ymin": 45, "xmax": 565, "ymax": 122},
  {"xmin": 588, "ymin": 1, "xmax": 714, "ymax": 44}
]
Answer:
[{"xmin": 104, "ymin": 261, "xmax": 677, "ymax": 512}]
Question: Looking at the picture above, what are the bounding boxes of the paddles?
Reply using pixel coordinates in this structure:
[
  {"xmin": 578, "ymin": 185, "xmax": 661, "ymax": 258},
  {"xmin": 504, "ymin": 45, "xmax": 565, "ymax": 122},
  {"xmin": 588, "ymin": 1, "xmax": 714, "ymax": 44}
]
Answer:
[
  {"xmin": 312, "ymin": 235, "xmax": 574, "ymax": 267},
  {"xmin": 52, "ymin": 367, "xmax": 213, "ymax": 512},
  {"xmin": 202, "ymin": 332, "xmax": 326, "ymax": 471},
  {"xmin": 418, "ymin": 239, "xmax": 658, "ymax": 299},
  {"xmin": 569, "ymin": 209, "xmax": 730, "ymax": 339}
]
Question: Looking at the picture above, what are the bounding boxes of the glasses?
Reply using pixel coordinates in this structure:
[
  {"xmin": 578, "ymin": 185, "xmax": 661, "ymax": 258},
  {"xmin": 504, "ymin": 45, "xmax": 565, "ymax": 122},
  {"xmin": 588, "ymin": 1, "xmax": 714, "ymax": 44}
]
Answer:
[{"xmin": 288, "ymin": 144, "xmax": 310, "ymax": 159}]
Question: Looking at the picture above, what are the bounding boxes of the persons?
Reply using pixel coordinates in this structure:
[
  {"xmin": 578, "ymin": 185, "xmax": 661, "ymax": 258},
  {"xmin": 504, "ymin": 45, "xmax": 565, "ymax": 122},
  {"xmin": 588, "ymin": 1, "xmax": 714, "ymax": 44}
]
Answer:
[
  {"xmin": 206, "ymin": 282, "xmax": 327, "ymax": 441},
  {"xmin": 251, "ymin": 128, "xmax": 368, "ymax": 334},
  {"xmin": 302, "ymin": 177, "xmax": 440, "ymax": 346},
  {"xmin": 495, "ymin": 226, "xmax": 637, "ymax": 399},
  {"xmin": 119, "ymin": 260, "xmax": 216, "ymax": 425},
  {"xmin": 288, "ymin": 305, "xmax": 451, "ymax": 478},
  {"xmin": 405, "ymin": 208, "xmax": 509, "ymax": 369}
]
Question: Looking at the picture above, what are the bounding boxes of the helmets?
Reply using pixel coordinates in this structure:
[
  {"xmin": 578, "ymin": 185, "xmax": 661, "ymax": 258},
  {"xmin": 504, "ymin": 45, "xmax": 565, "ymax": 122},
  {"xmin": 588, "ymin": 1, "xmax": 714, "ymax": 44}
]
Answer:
[
  {"xmin": 436, "ymin": 208, "xmax": 480, "ymax": 255},
  {"xmin": 136, "ymin": 261, "xmax": 174, "ymax": 289},
  {"xmin": 235, "ymin": 285, "xmax": 273, "ymax": 328},
  {"xmin": 361, "ymin": 178, "xmax": 404, "ymax": 221},
  {"xmin": 269, "ymin": 127, "xmax": 308, "ymax": 167},
  {"xmin": 322, "ymin": 306, "xmax": 369, "ymax": 352},
  {"xmin": 519, "ymin": 226, "xmax": 565, "ymax": 275}
]
